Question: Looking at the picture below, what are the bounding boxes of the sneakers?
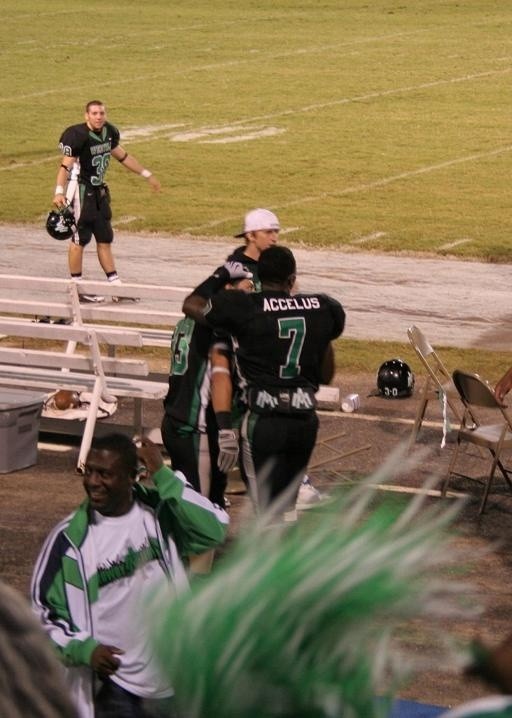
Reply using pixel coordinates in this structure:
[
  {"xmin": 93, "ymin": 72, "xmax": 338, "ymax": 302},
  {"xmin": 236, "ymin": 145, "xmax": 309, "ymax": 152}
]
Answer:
[
  {"xmin": 297, "ymin": 473, "xmax": 321, "ymax": 502},
  {"xmin": 78, "ymin": 294, "xmax": 142, "ymax": 305}
]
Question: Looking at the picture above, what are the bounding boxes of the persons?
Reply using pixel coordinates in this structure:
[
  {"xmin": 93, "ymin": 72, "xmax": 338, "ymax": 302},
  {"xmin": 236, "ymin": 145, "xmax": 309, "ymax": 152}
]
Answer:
[
  {"xmin": 223, "ymin": 208, "xmax": 281, "ymax": 290},
  {"xmin": 34, "ymin": 434, "xmax": 230, "ymax": 716},
  {"xmin": 163, "ymin": 264, "xmax": 255, "ymax": 503},
  {"xmin": 52, "ymin": 99, "xmax": 163, "ymax": 305},
  {"xmin": 181, "ymin": 245, "xmax": 347, "ymax": 530}
]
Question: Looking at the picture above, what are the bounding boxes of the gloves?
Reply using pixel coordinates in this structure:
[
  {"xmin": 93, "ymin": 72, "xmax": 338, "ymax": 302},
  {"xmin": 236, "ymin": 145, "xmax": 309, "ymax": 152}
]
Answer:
[
  {"xmin": 215, "ymin": 259, "xmax": 254, "ymax": 281},
  {"xmin": 216, "ymin": 428, "xmax": 240, "ymax": 475}
]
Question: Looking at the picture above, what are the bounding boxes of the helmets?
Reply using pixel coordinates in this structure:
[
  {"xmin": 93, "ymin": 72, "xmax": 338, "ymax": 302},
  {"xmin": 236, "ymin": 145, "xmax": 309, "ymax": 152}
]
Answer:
[
  {"xmin": 46, "ymin": 210, "xmax": 79, "ymax": 241},
  {"xmin": 377, "ymin": 356, "xmax": 415, "ymax": 399}
]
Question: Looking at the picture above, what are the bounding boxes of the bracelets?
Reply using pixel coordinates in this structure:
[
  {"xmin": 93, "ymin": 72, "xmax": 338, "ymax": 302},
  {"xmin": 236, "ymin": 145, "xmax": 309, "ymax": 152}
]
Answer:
[
  {"xmin": 139, "ymin": 168, "xmax": 155, "ymax": 179},
  {"xmin": 55, "ymin": 184, "xmax": 65, "ymax": 195}
]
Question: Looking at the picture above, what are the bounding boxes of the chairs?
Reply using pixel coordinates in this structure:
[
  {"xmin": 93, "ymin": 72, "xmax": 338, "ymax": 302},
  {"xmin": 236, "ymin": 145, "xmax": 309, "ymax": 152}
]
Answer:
[
  {"xmin": 407, "ymin": 327, "xmax": 485, "ymax": 458},
  {"xmin": 443, "ymin": 369, "xmax": 511, "ymax": 517}
]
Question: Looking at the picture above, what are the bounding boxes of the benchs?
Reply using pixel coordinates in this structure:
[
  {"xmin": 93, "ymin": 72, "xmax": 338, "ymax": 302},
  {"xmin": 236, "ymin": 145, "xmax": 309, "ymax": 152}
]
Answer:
[{"xmin": 0, "ymin": 272, "xmax": 197, "ymax": 478}]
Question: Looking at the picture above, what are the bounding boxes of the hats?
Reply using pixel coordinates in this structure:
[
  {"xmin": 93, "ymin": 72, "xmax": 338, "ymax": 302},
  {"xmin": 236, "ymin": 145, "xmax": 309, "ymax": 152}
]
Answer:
[{"xmin": 231, "ymin": 208, "xmax": 282, "ymax": 238}]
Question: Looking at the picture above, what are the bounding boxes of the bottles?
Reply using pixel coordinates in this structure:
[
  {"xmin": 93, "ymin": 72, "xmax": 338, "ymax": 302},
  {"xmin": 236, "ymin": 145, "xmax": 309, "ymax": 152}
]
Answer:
[{"xmin": 341, "ymin": 394, "xmax": 360, "ymax": 413}]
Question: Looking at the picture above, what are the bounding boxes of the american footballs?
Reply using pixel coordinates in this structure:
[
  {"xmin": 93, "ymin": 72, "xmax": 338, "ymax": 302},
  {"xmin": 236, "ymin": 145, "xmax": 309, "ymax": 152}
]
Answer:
[{"xmin": 54, "ymin": 390, "xmax": 81, "ymax": 408}]
